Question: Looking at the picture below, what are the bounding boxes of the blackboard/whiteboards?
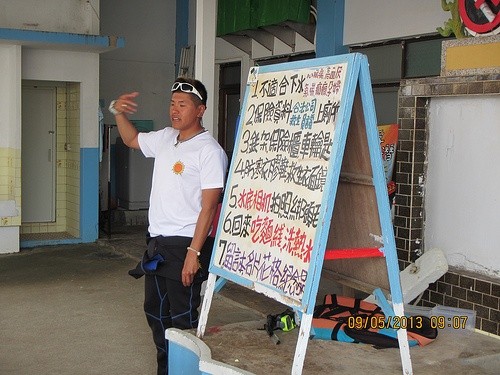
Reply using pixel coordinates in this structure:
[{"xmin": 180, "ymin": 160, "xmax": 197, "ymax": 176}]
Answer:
[{"xmin": 424, "ymin": 99, "xmax": 498, "ymax": 283}]
